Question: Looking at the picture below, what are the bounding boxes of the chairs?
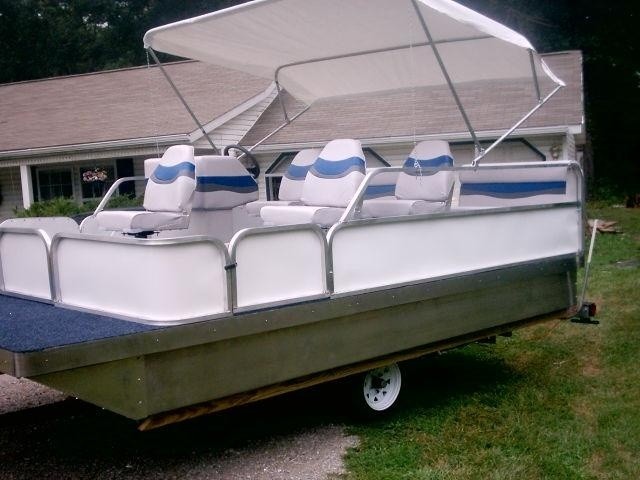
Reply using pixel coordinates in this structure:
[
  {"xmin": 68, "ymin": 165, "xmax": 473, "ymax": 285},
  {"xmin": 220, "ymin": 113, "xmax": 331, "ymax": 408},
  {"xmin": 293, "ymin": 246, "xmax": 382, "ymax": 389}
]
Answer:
[
  {"xmin": 97, "ymin": 145, "xmax": 196, "ymax": 238},
  {"xmin": 361, "ymin": 141, "xmax": 454, "ymax": 219},
  {"xmin": 260, "ymin": 139, "xmax": 367, "ymax": 230},
  {"xmin": 244, "ymin": 149, "xmax": 323, "ymax": 216}
]
